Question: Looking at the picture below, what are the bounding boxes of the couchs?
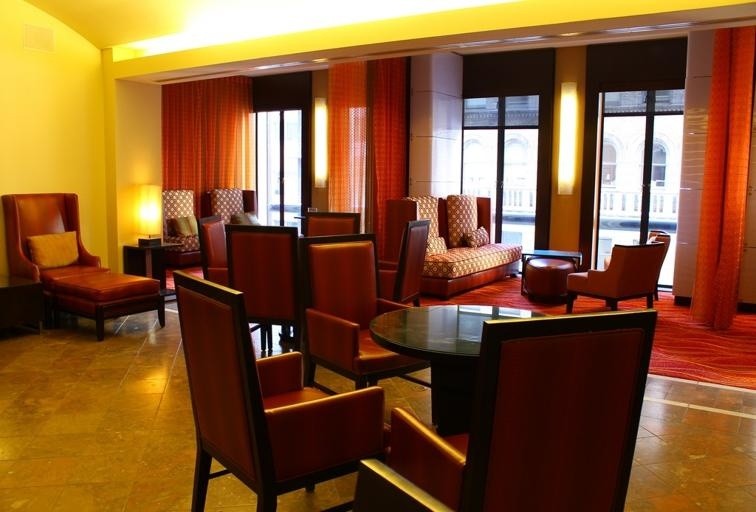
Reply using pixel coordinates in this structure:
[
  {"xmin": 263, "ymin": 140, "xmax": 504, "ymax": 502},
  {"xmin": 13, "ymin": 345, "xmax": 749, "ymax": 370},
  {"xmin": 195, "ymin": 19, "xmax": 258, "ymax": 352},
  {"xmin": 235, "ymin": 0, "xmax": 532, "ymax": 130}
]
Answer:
[
  {"xmin": 386, "ymin": 194, "xmax": 523, "ymax": 296},
  {"xmin": 160, "ymin": 189, "xmax": 257, "ymax": 265}
]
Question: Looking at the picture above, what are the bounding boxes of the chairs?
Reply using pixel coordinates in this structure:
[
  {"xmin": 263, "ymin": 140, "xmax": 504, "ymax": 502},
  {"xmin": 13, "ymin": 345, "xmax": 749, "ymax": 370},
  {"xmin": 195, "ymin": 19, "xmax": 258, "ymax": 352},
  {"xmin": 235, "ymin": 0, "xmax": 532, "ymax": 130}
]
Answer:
[
  {"xmin": 225, "ymin": 223, "xmax": 286, "ymax": 324},
  {"xmin": 563, "ymin": 239, "xmax": 669, "ymax": 314},
  {"xmin": 174, "ymin": 267, "xmax": 392, "ymax": 511},
  {"xmin": 297, "ymin": 231, "xmax": 438, "ymax": 423},
  {"xmin": 197, "ymin": 214, "xmax": 228, "ymax": 287},
  {"xmin": 604, "ymin": 229, "xmax": 672, "ymax": 300},
  {"xmin": 380, "ymin": 219, "xmax": 431, "ymax": 308},
  {"xmin": 381, "ymin": 307, "xmax": 659, "ymax": 512},
  {"xmin": 2, "ymin": 191, "xmax": 166, "ymax": 344},
  {"xmin": 304, "ymin": 211, "xmax": 361, "ymax": 239}
]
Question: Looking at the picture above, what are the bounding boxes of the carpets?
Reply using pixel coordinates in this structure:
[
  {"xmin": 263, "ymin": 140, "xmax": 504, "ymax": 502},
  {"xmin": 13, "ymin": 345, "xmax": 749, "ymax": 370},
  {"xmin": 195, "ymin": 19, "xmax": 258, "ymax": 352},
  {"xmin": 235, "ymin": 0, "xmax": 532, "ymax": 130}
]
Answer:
[{"xmin": 361, "ymin": 273, "xmax": 756, "ymax": 394}]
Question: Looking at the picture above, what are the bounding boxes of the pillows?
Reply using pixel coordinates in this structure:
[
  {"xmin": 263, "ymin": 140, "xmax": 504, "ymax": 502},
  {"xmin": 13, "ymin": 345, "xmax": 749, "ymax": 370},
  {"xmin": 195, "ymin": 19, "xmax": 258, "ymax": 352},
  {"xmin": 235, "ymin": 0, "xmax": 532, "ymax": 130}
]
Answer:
[
  {"xmin": 26, "ymin": 229, "xmax": 81, "ymax": 269},
  {"xmin": 465, "ymin": 226, "xmax": 490, "ymax": 248},
  {"xmin": 166, "ymin": 215, "xmax": 198, "ymax": 239},
  {"xmin": 230, "ymin": 210, "xmax": 261, "ymax": 228},
  {"xmin": 425, "ymin": 235, "xmax": 447, "ymax": 256}
]
